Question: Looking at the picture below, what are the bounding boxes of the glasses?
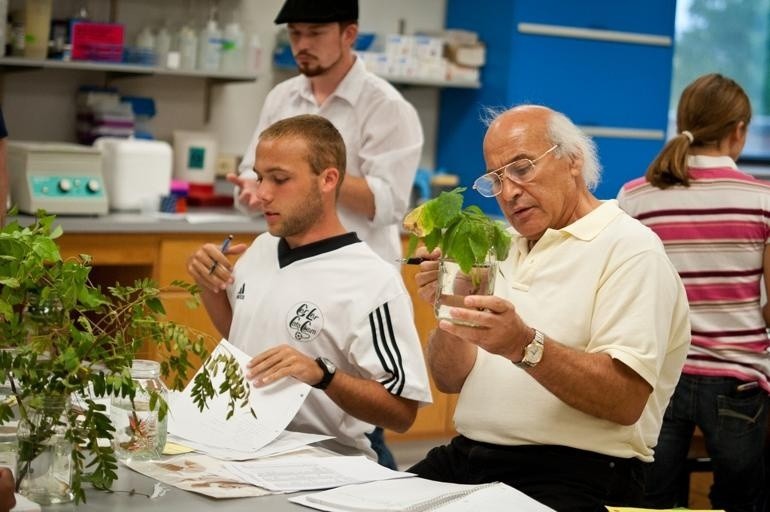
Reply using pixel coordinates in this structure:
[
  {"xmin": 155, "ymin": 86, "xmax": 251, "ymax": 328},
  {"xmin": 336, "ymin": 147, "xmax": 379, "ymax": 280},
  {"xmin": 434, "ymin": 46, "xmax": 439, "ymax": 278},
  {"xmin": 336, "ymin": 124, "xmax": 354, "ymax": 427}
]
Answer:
[{"xmin": 472, "ymin": 142, "xmax": 563, "ymax": 199}]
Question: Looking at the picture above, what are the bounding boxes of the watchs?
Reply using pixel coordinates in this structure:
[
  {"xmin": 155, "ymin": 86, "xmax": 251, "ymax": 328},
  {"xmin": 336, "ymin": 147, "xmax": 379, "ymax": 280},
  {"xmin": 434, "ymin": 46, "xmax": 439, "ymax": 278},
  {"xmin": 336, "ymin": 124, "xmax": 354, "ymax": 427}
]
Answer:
[
  {"xmin": 309, "ymin": 357, "xmax": 337, "ymax": 390},
  {"xmin": 510, "ymin": 329, "xmax": 546, "ymax": 369}
]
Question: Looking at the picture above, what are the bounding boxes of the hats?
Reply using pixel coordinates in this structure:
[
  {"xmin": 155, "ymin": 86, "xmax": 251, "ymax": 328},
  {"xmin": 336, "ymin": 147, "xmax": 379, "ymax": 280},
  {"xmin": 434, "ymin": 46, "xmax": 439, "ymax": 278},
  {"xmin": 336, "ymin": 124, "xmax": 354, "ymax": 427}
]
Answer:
[{"xmin": 274, "ymin": 1, "xmax": 358, "ymax": 23}]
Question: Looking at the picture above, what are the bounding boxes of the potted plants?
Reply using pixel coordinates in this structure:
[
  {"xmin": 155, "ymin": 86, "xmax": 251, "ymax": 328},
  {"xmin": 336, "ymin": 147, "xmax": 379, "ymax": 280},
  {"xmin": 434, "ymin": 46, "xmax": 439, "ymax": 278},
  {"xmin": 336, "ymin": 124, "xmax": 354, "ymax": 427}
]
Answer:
[
  {"xmin": 403, "ymin": 186, "xmax": 510, "ymax": 325},
  {"xmin": 0, "ymin": 202, "xmax": 258, "ymax": 505}
]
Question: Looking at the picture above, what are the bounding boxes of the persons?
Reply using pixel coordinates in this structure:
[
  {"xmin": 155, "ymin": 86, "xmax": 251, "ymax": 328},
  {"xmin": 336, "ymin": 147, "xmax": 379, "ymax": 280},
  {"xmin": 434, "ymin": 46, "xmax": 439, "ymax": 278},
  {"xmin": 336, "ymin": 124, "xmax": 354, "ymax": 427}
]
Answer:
[
  {"xmin": 225, "ymin": 0, "xmax": 425, "ymax": 471},
  {"xmin": 186, "ymin": 114, "xmax": 434, "ymax": 463},
  {"xmin": 0, "ymin": 466, "xmax": 16, "ymax": 512},
  {"xmin": 614, "ymin": 72, "xmax": 769, "ymax": 511},
  {"xmin": 405, "ymin": 104, "xmax": 691, "ymax": 511}
]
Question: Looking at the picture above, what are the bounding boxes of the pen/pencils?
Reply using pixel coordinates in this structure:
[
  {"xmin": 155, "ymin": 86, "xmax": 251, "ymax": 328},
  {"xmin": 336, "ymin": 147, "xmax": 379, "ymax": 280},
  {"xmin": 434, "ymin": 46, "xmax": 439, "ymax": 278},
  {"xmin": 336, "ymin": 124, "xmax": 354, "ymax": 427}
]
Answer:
[
  {"xmin": 208, "ymin": 234, "xmax": 233, "ymax": 276},
  {"xmin": 395, "ymin": 256, "xmax": 434, "ymax": 265}
]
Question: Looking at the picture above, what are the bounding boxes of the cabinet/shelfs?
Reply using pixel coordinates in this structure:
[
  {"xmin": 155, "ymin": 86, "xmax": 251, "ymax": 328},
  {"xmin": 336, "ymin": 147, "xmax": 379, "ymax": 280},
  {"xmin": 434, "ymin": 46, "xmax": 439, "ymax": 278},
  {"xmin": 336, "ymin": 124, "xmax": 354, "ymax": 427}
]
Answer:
[
  {"xmin": 0, "ymin": 207, "xmax": 269, "ymax": 391},
  {"xmin": 382, "ymin": 230, "xmax": 488, "ymax": 443}
]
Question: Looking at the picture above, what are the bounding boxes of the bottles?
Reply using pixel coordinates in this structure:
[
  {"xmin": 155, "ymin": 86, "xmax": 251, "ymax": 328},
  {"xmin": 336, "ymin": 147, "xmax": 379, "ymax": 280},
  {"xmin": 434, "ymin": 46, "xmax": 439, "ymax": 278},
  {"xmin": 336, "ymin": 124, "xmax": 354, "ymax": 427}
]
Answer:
[
  {"xmin": 6, "ymin": 9, "xmax": 25, "ymax": 57},
  {"xmin": 108, "ymin": 357, "xmax": 170, "ymax": 461},
  {"xmin": 137, "ymin": 16, "xmax": 264, "ymax": 75}
]
